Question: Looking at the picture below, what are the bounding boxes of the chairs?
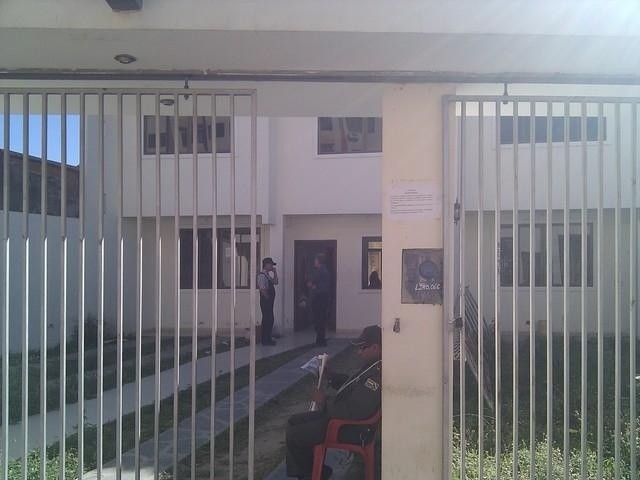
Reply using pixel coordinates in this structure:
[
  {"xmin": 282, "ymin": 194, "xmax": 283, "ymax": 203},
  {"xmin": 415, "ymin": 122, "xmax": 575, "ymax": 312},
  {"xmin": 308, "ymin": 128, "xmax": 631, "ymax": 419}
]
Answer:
[{"xmin": 312, "ymin": 407, "xmax": 381, "ymax": 480}]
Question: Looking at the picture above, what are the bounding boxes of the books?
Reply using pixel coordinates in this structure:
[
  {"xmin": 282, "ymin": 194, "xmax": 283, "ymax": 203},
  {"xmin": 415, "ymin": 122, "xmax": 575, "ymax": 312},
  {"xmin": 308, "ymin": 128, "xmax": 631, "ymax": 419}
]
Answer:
[{"xmin": 300, "ymin": 350, "xmax": 326, "ymax": 411}]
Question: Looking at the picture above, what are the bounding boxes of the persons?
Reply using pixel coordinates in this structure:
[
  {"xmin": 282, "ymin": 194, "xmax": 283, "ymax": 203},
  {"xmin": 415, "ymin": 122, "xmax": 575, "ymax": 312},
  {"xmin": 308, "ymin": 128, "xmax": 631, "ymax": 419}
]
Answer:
[
  {"xmin": 258, "ymin": 254, "xmax": 281, "ymax": 346},
  {"xmin": 280, "ymin": 324, "xmax": 380, "ymax": 479},
  {"xmin": 368, "ymin": 272, "xmax": 382, "ymax": 289},
  {"xmin": 302, "ymin": 252, "xmax": 331, "ymax": 349}
]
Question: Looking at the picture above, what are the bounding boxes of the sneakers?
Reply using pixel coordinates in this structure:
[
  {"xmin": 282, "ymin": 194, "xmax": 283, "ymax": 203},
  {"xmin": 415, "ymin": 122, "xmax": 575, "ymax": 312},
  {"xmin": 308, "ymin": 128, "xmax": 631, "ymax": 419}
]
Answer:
[{"xmin": 299, "ymin": 464, "xmax": 333, "ymax": 479}]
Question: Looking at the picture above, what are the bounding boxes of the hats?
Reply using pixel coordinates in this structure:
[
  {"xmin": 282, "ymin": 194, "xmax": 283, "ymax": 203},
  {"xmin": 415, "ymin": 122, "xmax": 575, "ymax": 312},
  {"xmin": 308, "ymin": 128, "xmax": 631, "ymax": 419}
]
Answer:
[
  {"xmin": 349, "ymin": 325, "xmax": 381, "ymax": 347},
  {"xmin": 263, "ymin": 257, "xmax": 276, "ymax": 266}
]
